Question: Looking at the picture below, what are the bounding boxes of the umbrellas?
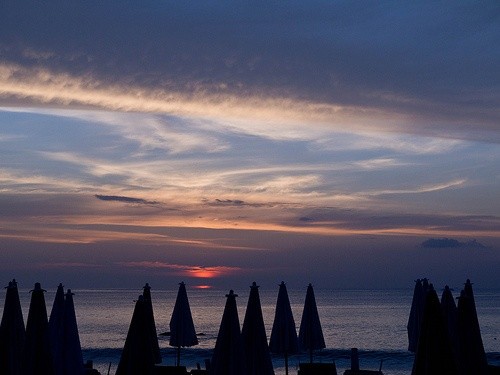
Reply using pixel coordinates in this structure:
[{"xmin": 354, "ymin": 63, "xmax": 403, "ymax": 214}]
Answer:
[
  {"xmin": 240, "ymin": 282, "xmax": 276, "ymax": 375},
  {"xmin": 142, "ymin": 283, "xmax": 163, "ymax": 364},
  {"xmin": 64, "ymin": 288, "xmax": 87, "ymax": 375},
  {"xmin": 18, "ymin": 283, "xmax": 54, "ymax": 375},
  {"xmin": 269, "ymin": 281, "xmax": 300, "ymax": 375},
  {"xmin": 48, "ymin": 283, "xmax": 74, "ymax": 375},
  {"xmin": 406, "ymin": 278, "xmax": 492, "ymax": 375},
  {"xmin": 115, "ymin": 295, "xmax": 155, "ymax": 375},
  {"xmin": 0, "ymin": 279, "xmax": 25, "ymax": 375},
  {"xmin": 298, "ymin": 284, "xmax": 326, "ymax": 363},
  {"xmin": 168, "ymin": 282, "xmax": 199, "ymax": 365},
  {"xmin": 209, "ymin": 288, "xmax": 249, "ymax": 375}
]
